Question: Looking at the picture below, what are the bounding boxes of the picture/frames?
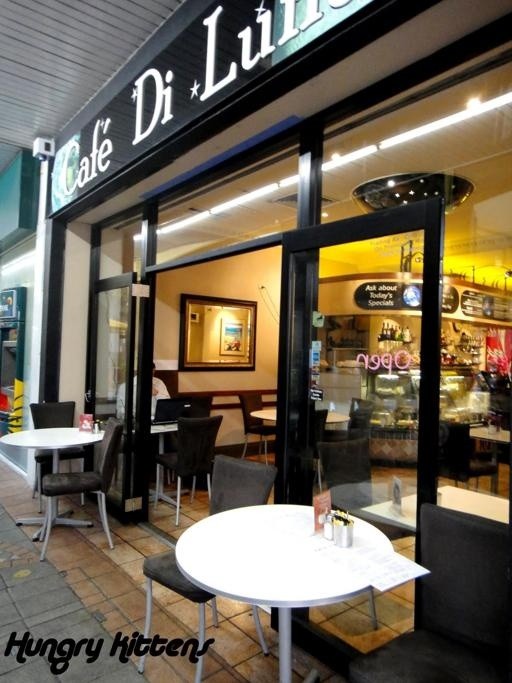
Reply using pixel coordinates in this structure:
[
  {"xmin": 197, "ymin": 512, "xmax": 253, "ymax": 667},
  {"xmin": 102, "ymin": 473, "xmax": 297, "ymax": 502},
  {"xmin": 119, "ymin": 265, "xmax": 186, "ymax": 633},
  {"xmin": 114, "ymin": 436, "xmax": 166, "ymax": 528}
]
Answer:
[{"xmin": 219, "ymin": 318, "xmax": 245, "ymax": 357}]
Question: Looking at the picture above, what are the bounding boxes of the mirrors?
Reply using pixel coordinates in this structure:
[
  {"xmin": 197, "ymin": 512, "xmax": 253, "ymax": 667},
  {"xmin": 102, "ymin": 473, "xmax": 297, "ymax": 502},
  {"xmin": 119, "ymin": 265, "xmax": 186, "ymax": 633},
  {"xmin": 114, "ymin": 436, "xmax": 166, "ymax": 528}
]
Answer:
[{"xmin": 177, "ymin": 292, "xmax": 258, "ymax": 372}]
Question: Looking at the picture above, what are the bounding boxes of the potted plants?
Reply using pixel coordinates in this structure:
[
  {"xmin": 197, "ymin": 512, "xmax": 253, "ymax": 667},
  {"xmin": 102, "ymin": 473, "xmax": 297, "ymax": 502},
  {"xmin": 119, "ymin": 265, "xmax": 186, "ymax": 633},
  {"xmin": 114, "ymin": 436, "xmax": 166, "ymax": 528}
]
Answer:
[{"xmin": 219, "ymin": 318, "xmax": 245, "ymax": 357}]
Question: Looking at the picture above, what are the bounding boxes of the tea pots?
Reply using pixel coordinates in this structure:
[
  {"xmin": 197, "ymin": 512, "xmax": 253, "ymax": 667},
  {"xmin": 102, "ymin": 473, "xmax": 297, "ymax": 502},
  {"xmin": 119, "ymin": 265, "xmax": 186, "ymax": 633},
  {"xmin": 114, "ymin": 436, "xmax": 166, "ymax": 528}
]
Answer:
[{"xmin": 177, "ymin": 292, "xmax": 258, "ymax": 372}]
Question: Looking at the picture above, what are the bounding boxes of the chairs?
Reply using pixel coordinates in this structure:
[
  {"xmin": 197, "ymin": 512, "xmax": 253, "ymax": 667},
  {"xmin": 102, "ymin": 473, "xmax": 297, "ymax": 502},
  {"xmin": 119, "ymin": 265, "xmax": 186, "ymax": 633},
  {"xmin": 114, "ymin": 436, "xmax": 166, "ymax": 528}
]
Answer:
[
  {"xmin": 313, "ymin": 394, "xmax": 510, "ymax": 681},
  {"xmin": 27, "ymin": 387, "xmax": 275, "ymax": 682}
]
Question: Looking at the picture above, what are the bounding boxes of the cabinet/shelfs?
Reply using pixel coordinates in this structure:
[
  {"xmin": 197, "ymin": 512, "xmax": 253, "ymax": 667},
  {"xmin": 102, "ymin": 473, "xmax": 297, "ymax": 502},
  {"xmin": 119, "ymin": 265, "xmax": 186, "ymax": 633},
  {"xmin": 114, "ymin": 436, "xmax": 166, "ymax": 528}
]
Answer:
[
  {"xmin": 379, "ymin": 323, "xmax": 410, "ymax": 341},
  {"xmin": 324, "ymin": 516, "xmax": 334, "ymax": 541},
  {"xmin": 93, "ymin": 423, "xmax": 100, "ymax": 434}
]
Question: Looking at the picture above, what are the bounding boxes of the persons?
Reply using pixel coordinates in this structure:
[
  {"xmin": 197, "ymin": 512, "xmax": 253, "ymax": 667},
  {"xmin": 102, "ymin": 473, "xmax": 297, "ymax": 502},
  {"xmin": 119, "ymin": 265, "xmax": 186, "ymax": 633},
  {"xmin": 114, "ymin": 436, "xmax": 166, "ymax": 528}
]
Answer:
[{"xmin": 117, "ymin": 362, "xmax": 171, "ymax": 418}]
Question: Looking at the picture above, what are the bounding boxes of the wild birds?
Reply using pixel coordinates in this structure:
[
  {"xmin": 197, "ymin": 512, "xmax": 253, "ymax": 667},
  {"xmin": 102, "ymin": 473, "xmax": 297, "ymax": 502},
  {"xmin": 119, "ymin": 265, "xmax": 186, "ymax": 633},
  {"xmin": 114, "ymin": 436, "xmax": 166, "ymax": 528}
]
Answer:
[{"xmin": 151, "ymin": 396, "xmax": 193, "ymax": 423}]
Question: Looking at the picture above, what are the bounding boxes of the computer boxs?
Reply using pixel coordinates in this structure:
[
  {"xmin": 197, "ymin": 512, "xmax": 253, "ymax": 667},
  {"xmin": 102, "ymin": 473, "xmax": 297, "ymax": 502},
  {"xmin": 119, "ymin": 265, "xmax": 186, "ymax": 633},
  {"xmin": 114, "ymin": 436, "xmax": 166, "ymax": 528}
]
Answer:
[{"xmin": 32, "ymin": 137, "xmax": 55, "ymax": 160}]
